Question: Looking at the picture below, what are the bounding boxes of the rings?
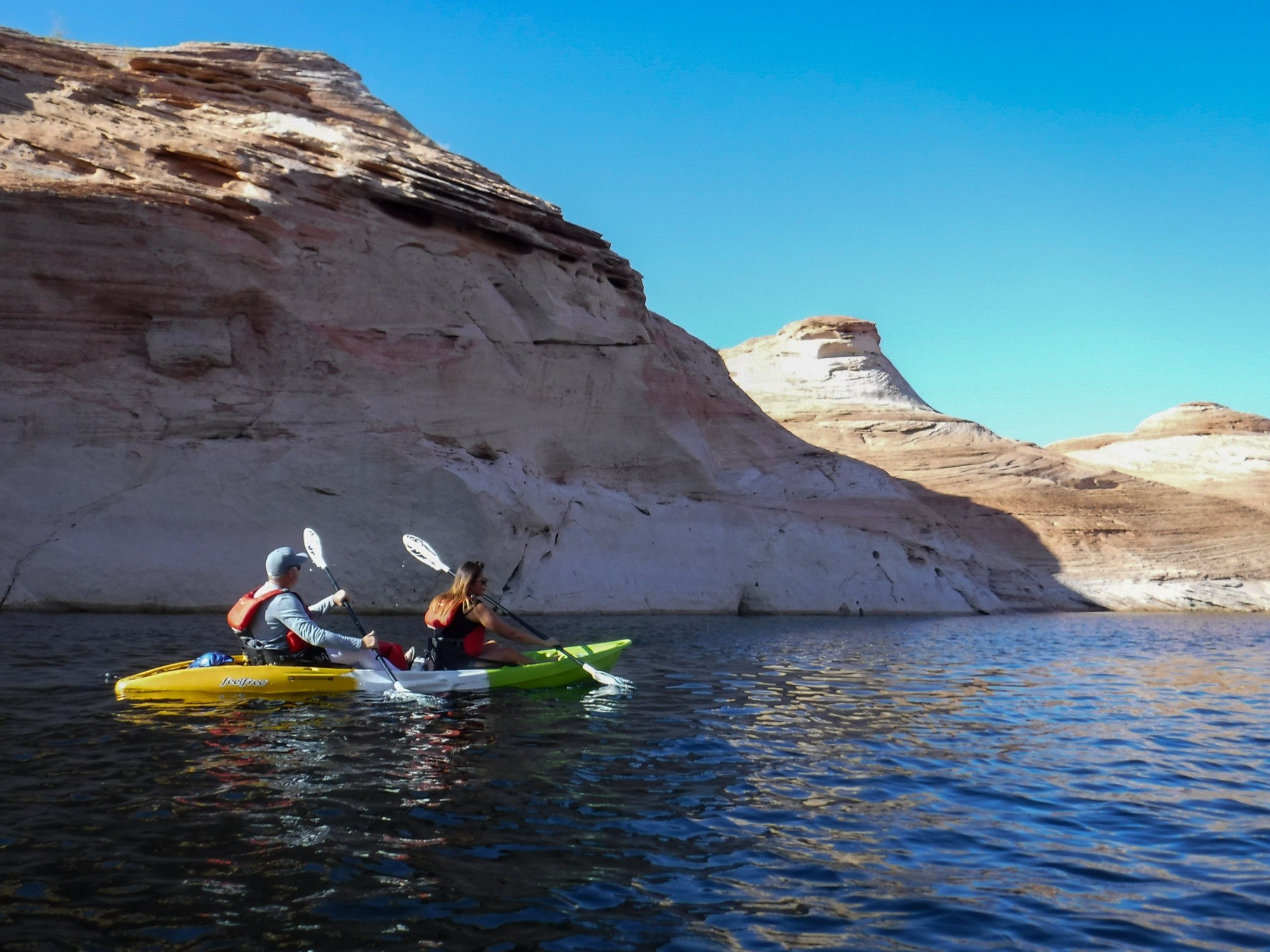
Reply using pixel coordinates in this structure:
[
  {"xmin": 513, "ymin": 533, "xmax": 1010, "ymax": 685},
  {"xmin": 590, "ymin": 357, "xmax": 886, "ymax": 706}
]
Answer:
[{"xmin": 556, "ymin": 640, "xmax": 558, "ymax": 643}]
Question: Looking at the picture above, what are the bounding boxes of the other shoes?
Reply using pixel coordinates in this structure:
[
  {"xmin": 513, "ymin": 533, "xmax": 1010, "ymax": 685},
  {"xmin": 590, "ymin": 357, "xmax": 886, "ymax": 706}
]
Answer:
[{"xmin": 403, "ymin": 647, "xmax": 416, "ymax": 670}]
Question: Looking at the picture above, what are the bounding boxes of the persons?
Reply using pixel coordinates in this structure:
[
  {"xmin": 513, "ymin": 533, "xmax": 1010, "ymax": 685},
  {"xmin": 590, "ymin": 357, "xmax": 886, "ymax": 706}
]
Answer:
[
  {"xmin": 227, "ymin": 546, "xmax": 436, "ymax": 671},
  {"xmin": 424, "ymin": 560, "xmax": 563, "ymax": 671}
]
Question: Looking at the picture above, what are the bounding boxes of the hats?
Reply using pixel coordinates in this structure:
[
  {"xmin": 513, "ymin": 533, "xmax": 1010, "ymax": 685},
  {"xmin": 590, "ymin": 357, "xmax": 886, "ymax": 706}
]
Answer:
[{"xmin": 265, "ymin": 546, "xmax": 308, "ymax": 577}]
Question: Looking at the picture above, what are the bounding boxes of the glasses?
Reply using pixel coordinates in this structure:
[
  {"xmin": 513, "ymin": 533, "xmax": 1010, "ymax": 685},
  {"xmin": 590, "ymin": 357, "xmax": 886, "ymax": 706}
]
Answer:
[
  {"xmin": 474, "ymin": 577, "xmax": 487, "ymax": 585},
  {"xmin": 287, "ymin": 565, "xmax": 301, "ymax": 572}
]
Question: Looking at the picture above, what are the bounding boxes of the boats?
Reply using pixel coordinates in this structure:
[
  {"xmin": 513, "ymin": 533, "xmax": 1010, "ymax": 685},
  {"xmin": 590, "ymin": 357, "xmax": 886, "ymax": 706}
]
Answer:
[{"xmin": 114, "ymin": 637, "xmax": 633, "ymax": 703}]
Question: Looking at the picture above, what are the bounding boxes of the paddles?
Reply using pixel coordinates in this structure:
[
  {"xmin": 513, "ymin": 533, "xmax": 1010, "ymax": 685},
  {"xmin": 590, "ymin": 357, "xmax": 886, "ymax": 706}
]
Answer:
[
  {"xmin": 303, "ymin": 527, "xmax": 413, "ymax": 693},
  {"xmin": 402, "ymin": 533, "xmax": 613, "ymax": 684}
]
